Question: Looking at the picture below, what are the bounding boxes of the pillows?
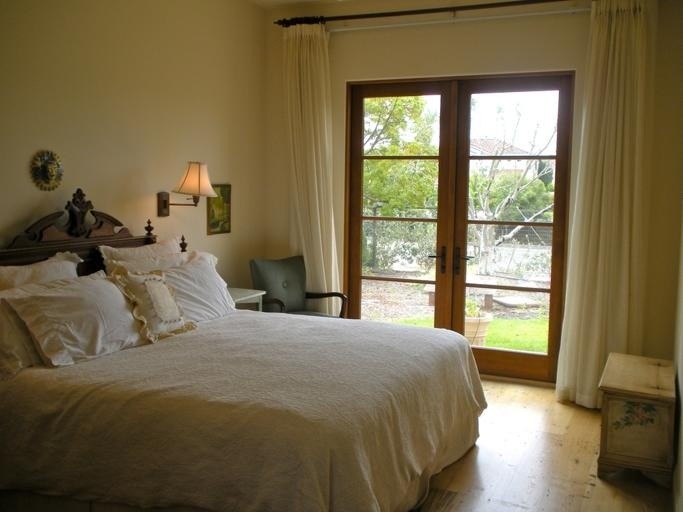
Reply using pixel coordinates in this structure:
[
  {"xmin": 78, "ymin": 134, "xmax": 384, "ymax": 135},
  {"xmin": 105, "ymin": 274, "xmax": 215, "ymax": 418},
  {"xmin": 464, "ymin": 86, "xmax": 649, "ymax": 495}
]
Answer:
[{"xmin": 0, "ymin": 234, "xmax": 237, "ymax": 381}]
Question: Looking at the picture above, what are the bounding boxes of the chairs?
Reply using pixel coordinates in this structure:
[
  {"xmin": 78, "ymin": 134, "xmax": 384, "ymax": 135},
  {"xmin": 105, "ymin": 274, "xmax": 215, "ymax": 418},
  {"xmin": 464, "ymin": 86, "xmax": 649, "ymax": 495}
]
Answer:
[{"xmin": 249, "ymin": 255, "xmax": 348, "ymax": 319}]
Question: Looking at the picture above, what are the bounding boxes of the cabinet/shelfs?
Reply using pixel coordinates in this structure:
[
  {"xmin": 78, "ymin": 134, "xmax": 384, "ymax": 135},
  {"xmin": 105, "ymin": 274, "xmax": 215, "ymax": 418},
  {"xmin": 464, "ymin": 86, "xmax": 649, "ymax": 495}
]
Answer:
[{"xmin": 597, "ymin": 352, "xmax": 677, "ymax": 489}]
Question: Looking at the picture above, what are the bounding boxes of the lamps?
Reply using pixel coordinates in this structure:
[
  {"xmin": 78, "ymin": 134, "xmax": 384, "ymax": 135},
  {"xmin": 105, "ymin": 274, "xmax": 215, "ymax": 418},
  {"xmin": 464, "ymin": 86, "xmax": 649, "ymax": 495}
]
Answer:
[{"xmin": 157, "ymin": 161, "xmax": 218, "ymax": 217}]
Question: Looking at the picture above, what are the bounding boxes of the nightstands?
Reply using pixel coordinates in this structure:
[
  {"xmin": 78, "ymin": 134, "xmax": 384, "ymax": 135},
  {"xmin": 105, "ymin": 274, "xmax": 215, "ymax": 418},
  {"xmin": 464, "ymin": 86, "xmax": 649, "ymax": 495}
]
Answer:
[{"xmin": 228, "ymin": 288, "xmax": 266, "ymax": 311}]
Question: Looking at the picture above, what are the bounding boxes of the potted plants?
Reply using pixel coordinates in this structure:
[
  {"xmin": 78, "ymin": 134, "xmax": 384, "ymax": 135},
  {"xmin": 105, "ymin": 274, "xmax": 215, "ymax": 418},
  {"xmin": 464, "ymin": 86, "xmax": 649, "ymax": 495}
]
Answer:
[{"xmin": 465, "ymin": 299, "xmax": 491, "ymax": 346}]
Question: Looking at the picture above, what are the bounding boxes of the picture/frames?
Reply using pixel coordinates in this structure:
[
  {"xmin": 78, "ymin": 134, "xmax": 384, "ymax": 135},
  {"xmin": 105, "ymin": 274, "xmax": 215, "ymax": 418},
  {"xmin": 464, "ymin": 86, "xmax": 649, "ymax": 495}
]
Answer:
[{"xmin": 207, "ymin": 184, "xmax": 232, "ymax": 234}]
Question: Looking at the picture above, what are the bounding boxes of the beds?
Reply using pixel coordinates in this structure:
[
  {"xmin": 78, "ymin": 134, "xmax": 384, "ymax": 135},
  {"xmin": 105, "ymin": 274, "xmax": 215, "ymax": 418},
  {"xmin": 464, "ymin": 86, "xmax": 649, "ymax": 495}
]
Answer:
[{"xmin": 0, "ymin": 188, "xmax": 488, "ymax": 512}]
